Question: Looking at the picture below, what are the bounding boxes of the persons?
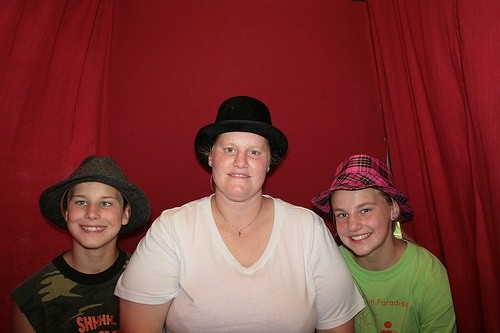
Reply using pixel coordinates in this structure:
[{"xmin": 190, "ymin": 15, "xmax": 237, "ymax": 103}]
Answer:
[
  {"xmin": 310, "ymin": 154, "xmax": 457, "ymax": 333},
  {"xmin": 11, "ymin": 156, "xmax": 150, "ymax": 333},
  {"xmin": 114, "ymin": 96, "xmax": 366, "ymax": 333}
]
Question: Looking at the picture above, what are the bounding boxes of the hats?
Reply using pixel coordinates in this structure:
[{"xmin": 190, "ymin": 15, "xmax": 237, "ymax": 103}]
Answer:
[
  {"xmin": 193, "ymin": 95, "xmax": 289, "ymax": 178},
  {"xmin": 38, "ymin": 155, "xmax": 151, "ymax": 238},
  {"xmin": 311, "ymin": 155, "xmax": 415, "ymax": 225}
]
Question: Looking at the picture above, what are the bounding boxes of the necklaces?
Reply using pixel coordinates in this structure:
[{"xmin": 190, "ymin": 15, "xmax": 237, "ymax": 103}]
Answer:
[{"xmin": 215, "ymin": 196, "xmax": 262, "ymax": 237}]
